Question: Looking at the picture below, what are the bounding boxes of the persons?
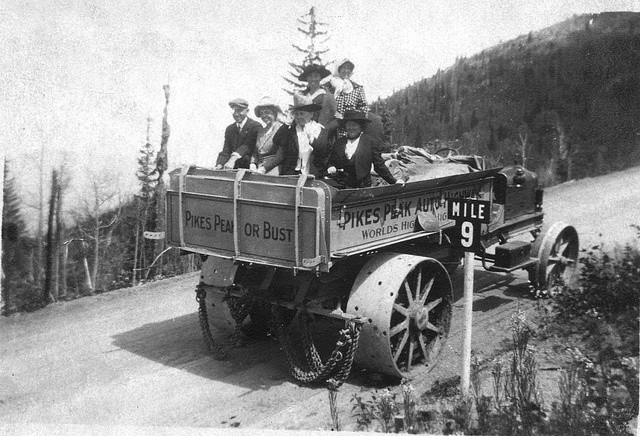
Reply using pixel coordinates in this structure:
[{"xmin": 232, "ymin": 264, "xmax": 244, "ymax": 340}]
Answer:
[
  {"xmin": 319, "ymin": 108, "xmax": 405, "ymax": 190},
  {"xmin": 294, "ymin": 63, "xmax": 337, "ymax": 127},
  {"xmin": 213, "ymin": 99, "xmax": 263, "ymax": 170},
  {"xmin": 281, "ymin": 94, "xmax": 326, "ymax": 175},
  {"xmin": 320, "ymin": 57, "xmax": 370, "ymax": 119},
  {"xmin": 249, "ymin": 96, "xmax": 289, "ymax": 179}
]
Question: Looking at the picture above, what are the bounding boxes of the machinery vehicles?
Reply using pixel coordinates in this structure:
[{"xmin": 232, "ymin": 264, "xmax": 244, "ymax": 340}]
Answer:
[{"xmin": 165, "ymin": 145, "xmax": 580, "ymax": 392}]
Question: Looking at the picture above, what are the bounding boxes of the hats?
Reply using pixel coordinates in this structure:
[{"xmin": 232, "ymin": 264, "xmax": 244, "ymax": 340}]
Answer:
[
  {"xmin": 289, "ymin": 95, "xmax": 322, "ymax": 112},
  {"xmin": 229, "ymin": 99, "xmax": 248, "ymax": 108},
  {"xmin": 254, "ymin": 97, "xmax": 281, "ymax": 117},
  {"xmin": 340, "ymin": 110, "xmax": 372, "ymax": 125},
  {"xmin": 298, "ymin": 64, "xmax": 331, "ymax": 81}
]
